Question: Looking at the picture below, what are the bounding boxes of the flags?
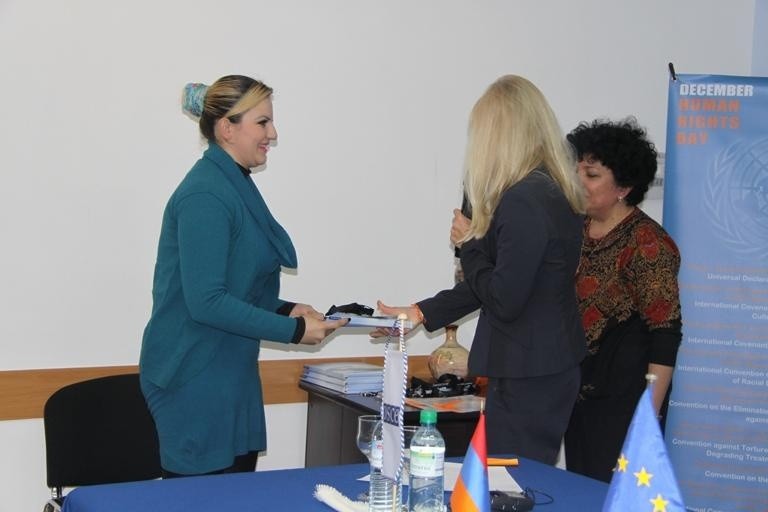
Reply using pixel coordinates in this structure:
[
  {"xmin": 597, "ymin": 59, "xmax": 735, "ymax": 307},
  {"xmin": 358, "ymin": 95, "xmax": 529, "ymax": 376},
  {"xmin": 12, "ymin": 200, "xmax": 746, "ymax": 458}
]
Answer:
[
  {"xmin": 449, "ymin": 412, "xmax": 491, "ymax": 512},
  {"xmin": 601, "ymin": 384, "xmax": 686, "ymax": 512}
]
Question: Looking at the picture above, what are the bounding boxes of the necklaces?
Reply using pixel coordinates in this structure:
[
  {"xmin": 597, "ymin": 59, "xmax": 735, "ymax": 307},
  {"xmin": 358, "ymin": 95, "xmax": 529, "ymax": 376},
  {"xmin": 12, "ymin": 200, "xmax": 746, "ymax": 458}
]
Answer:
[{"xmin": 574, "ymin": 208, "xmax": 627, "ymax": 274}]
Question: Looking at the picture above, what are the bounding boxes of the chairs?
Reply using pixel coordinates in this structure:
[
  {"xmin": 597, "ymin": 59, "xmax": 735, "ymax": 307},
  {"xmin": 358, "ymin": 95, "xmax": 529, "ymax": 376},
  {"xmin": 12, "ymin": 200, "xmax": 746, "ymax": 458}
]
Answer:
[{"xmin": 44, "ymin": 373, "xmax": 162, "ymax": 512}]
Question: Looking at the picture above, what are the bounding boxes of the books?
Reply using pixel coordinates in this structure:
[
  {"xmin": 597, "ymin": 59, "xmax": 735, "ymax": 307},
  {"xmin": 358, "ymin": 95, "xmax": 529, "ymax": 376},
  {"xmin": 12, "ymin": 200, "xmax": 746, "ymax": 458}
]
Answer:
[
  {"xmin": 303, "ymin": 361, "xmax": 385, "ymax": 380},
  {"xmin": 304, "ymin": 370, "xmax": 383, "ymax": 387},
  {"xmin": 303, "ymin": 375, "xmax": 382, "ymax": 395},
  {"xmin": 327, "ymin": 312, "xmax": 414, "ymax": 330}
]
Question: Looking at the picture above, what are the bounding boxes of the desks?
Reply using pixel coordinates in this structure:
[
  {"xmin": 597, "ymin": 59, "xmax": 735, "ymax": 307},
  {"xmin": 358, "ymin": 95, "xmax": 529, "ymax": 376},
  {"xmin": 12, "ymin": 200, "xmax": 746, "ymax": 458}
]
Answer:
[{"xmin": 66, "ymin": 453, "xmax": 611, "ymax": 512}]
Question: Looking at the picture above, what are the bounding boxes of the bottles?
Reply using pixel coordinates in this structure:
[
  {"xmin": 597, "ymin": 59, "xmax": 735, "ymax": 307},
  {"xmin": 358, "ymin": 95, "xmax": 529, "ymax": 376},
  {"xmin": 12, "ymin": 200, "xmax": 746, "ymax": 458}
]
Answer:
[
  {"xmin": 409, "ymin": 409, "xmax": 446, "ymax": 512},
  {"xmin": 370, "ymin": 421, "xmax": 403, "ymax": 511}
]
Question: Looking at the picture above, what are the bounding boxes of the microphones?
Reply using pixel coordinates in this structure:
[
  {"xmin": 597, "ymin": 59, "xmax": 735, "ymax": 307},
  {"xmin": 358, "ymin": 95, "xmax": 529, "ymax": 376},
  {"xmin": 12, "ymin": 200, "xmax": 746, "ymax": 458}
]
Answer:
[{"xmin": 455, "ymin": 191, "xmax": 473, "ymax": 260}]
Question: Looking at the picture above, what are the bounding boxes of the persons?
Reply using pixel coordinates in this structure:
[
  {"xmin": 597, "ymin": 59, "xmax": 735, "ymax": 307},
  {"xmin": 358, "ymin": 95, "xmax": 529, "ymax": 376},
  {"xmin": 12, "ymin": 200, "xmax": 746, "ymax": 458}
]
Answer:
[
  {"xmin": 563, "ymin": 111, "xmax": 684, "ymax": 485},
  {"xmin": 367, "ymin": 75, "xmax": 593, "ymax": 468},
  {"xmin": 137, "ymin": 74, "xmax": 353, "ymax": 479}
]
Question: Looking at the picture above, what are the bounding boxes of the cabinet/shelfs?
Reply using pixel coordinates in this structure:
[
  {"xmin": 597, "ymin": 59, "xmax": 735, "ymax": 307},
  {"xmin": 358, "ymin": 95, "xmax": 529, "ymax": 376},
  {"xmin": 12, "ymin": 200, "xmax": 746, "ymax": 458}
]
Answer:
[{"xmin": 298, "ymin": 379, "xmax": 482, "ymax": 467}]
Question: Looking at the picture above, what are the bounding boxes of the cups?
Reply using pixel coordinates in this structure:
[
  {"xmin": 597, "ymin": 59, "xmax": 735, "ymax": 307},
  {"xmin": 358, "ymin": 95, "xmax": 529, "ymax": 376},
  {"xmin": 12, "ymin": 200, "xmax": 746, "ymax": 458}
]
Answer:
[
  {"xmin": 356, "ymin": 415, "xmax": 382, "ymax": 463},
  {"xmin": 401, "ymin": 424, "xmax": 422, "ymax": 473}
]
